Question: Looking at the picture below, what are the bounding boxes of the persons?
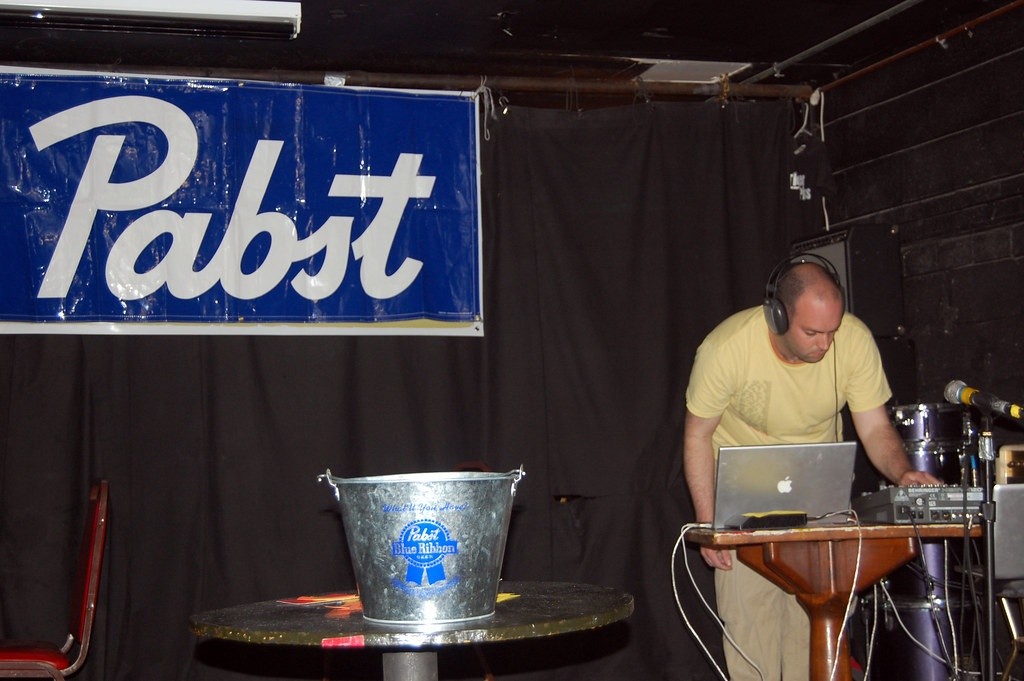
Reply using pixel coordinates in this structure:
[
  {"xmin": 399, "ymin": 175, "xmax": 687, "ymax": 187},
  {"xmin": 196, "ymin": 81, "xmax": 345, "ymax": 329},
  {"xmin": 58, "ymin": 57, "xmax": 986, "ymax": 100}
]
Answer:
[{"xmin": 683, "ymin": 262, "xmax": 943, "ymax": 681}]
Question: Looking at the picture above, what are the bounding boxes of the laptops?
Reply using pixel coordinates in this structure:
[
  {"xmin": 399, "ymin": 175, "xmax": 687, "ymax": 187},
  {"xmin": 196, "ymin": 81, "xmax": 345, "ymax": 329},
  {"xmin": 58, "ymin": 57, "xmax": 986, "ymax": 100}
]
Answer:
[{"xmin": 711, "ymin": 441, "xmax": 858, "ymax": 531}]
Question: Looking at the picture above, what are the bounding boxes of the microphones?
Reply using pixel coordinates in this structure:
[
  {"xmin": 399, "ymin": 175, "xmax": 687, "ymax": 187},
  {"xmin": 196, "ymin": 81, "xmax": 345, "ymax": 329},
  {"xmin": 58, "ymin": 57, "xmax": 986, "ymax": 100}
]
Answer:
[{"xmin": 944, "ymin": 379, "xmax": 1024, "ymax": 418}]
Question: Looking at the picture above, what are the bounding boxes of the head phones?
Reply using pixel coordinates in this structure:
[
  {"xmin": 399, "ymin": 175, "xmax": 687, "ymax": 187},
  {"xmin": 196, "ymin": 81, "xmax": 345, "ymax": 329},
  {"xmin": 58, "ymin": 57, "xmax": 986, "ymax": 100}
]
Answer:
[{"xmin": 762, "ymin": 252, "xmax": 846, "ymax": 336}]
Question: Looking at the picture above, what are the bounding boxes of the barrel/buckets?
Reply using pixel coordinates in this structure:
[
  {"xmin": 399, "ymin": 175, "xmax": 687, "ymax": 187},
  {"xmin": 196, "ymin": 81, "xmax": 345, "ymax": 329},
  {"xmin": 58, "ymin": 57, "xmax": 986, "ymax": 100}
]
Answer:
[{"xmin": 318, "ymin": 464, "xmax": 527, "ymax": 625}]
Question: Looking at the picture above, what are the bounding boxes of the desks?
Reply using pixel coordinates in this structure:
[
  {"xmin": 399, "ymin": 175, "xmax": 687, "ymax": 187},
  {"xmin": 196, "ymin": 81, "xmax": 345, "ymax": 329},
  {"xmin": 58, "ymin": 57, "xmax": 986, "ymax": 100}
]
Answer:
[
  {"xmin": 683, "ymin": 524, "xmax": 982, "ymax": 681},
  {"xmin": 186, "ymin": 582, "xmax": 635, "ymax": 681}
]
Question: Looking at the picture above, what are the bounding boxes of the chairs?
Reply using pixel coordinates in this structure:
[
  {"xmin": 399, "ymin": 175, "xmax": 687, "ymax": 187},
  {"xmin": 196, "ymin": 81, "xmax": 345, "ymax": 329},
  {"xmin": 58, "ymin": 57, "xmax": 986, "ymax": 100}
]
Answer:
[
  {"xmin": 0, "ymin": 482, "xmax": 108, "ymax": 681},
  {"xmin": 954, "ymin": 484, "xmax": 1024, "ymax": 681}
]
